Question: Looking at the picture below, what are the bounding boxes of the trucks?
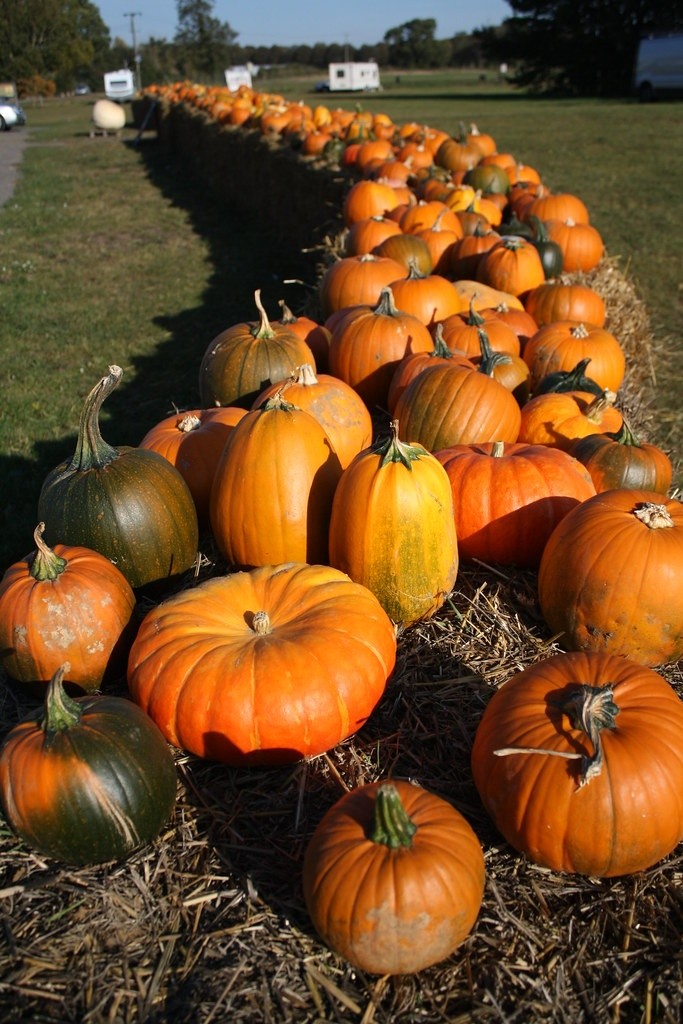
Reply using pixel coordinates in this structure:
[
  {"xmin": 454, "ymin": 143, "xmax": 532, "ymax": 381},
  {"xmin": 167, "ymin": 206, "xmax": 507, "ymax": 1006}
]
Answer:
[{"xmin": 328, "ymin": 62, "xmax": 379, "ymax": 90}]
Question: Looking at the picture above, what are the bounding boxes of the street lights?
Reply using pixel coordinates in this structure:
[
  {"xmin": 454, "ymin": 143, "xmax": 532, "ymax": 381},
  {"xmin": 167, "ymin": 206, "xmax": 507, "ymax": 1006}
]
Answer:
[{"xmin": 123, "ymin": 11, "xmax": 143, "ymax": 91}]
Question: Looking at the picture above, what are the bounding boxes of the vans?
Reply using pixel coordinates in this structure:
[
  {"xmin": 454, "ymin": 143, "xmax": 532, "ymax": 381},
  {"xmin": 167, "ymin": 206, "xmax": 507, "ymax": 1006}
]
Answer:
[{"xmin": 104, "ymin": 70, "xmax": 136, "ymax": 100}]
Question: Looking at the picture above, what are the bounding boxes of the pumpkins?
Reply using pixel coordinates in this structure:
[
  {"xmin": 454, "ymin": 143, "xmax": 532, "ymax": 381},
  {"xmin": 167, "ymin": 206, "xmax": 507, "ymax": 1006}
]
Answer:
[{"xmin": 0, "ymin": 82, "xmax": 683, "ymax": 976}]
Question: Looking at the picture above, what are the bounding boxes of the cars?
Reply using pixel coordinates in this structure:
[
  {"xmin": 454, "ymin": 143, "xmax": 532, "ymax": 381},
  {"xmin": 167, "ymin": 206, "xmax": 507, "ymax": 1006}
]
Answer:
[
  {"xmin": 0, "ymin": 101, "xmax": 26, "ymax": 131},
  {"xmin": 76, "ymin": 85, "xmax": 89, "ymax": 96},
  {"xmin": 315, "ymin": 81, "xmax": 330, "ymax": 92}
]
